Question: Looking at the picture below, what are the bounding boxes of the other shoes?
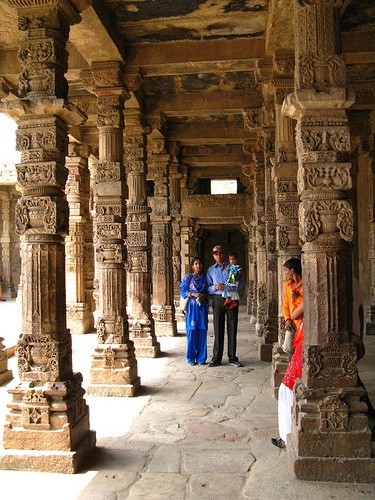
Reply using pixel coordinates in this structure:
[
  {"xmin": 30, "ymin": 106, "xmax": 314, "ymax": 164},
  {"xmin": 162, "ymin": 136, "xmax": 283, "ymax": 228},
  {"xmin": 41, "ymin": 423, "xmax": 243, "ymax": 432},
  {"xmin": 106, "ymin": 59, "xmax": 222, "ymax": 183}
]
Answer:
[
  {"xmin": 271, "ymin": 437, "xmax": 287, "ymax": 448},
  {"xmin": 208, "ymin": 361, "xmax": 221, "ymax": 366},
  {"xmin": 224, "ymin": 298, "xmax": 231, "ymax": 306},
  {"xmin": 188, "ymin": 362, "xmax": 203, "ymax": 366},
  {"xmin": 229, "ymin": 302, "xmax": 237, "ymax": 309},
  {"xmin": 229, "ymin": 360, "xmax": 241, "ymax": 367}
]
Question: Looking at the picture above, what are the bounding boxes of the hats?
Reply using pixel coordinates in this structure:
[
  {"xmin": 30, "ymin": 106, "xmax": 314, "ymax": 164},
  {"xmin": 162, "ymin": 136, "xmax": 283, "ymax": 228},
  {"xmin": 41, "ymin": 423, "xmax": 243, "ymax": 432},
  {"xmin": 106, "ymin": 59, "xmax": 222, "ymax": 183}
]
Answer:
[{"xmin": 213, "ymin": 245, "xmax": 224, "ymax": 254}]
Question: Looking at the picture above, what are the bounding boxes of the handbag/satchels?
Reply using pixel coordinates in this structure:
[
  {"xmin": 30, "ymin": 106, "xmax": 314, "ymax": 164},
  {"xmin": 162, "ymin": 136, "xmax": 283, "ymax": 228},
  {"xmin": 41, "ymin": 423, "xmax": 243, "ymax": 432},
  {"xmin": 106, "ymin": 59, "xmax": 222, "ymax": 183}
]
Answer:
[{"xmin": 180, "ymin": 296, "xmax": 189, "ymax": 311}]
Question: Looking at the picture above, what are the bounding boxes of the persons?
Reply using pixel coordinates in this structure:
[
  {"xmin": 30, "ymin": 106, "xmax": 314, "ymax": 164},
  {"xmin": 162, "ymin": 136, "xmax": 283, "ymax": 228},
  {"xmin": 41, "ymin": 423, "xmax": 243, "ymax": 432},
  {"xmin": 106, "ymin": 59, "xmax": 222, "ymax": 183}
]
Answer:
[
  {"xmin": 179, "ymin": 256, "xmax": 210, "ymax": 365},
  {"xmin": 271, "ymin": 300, "xmax": 305, "ymax": 448},
  {"xmin": 207, "ymin": 246, "xmax": 245, "ymax": 367},
  {"xmin": 276, "ymin": 258, "xmax": 364, "ymax": 339},
  {"xmin": 222, "ymin": 252, "xmax": 242, "ymax": 309}
]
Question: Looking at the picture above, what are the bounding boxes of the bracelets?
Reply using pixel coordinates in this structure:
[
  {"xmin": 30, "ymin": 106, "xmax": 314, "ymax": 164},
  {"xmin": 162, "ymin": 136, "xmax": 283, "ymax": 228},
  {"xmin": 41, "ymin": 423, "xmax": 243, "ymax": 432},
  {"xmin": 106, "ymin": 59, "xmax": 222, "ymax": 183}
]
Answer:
[
  {"xmin": 188, "ymin": 292, "xmax": 191, "ymax": 297},
  {"xmin": 285, "ymin": 318, "xmax": 291, "ymax": 322}
]
